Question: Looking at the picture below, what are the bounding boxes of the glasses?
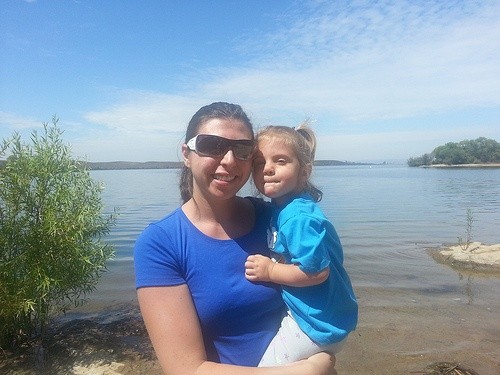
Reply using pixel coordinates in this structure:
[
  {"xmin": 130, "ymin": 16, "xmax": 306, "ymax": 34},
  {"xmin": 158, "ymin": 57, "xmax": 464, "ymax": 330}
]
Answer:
[{"xmin": 186, "ymin": 134, "xmax": 254, "ymax": 161}]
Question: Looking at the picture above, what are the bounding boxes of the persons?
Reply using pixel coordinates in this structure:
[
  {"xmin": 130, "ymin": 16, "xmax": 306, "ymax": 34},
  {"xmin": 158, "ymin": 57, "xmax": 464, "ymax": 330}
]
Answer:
[
  {"xmin": 244, "ymin": 121, "xmax": 358, "ymax": 368},
  {"xmin": 134, "ymin": 101, "xmax": 338, "ymax": 375}
]
